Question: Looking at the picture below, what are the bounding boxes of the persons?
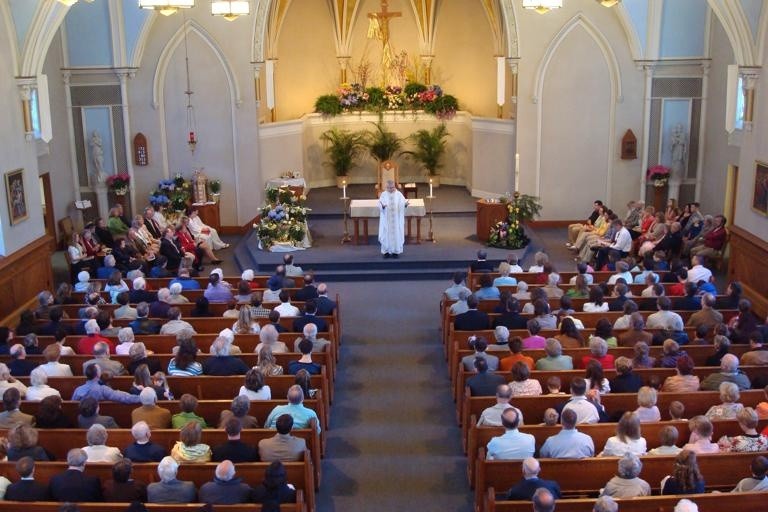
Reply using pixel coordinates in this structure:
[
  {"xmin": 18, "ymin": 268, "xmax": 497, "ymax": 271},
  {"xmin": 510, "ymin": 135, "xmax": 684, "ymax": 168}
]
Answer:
[
  {"xmin": 670, "ymin": 124, "xmax": 687, "ymax": 181},
  {"xmin": 566, "ymin": 198, "xmax": 729, "ymax": 267},
  {"xmin": 0, "ymin": 254, "xmax": 337, "ymax": 512},
  {"xmin": 9, "ymin": 175, "xmax": 27, "ymax": 220},
  {"xmin": 66, "ymin": 202, "xmax": 231, "ymax": 278},
  {"xmin": 378, "ymin": 180, "xmax": 410, "ymax": 258},
  {"xmin": 89, "ymin": 131, "xmax": 109, "ymax": 186},
  {"xmin": 443, "ymin": 255, "xmax": 768, "ymax": 510}
]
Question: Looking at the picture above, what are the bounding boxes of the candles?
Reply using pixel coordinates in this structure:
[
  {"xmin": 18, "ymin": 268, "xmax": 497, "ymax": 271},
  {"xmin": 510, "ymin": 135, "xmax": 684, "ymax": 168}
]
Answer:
[
  {"xmin": 343, "ymin": 184, "xmax": 346, "ymax": 198},
  {"xmin": 429, "ymin": 183, "xmax": 432, "ymax": 196}
]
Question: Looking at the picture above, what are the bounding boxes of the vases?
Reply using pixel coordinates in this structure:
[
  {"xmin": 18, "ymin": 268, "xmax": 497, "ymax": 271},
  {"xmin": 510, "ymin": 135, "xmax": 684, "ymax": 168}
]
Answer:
[
  {"xmin": 654, "ymin": 178, "xmax": 666, "ymax": 187},
  {"xmin": 114, "ymin": 185, "xmax": 128, "ymax": 196}
]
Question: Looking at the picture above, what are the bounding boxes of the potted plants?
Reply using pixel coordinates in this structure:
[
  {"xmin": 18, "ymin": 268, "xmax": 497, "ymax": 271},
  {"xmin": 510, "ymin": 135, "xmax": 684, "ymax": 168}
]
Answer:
[
  {"xmin": 320, "ymin": 129, "xmax": 366, "ymax": 191},
  {"xmin": 210, "ymin": 180, "xmax": 221, "ymax": 204},
  {"xmin": 405, "ymin": 122, "xmax": 453, "ymax": 188}
]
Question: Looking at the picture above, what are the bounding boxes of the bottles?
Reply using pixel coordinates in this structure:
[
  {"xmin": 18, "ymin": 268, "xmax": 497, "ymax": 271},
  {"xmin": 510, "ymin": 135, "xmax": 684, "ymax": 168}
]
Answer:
[{"xmin": 169, "ymin": 391, "xmax": 173, "ymax": 400}]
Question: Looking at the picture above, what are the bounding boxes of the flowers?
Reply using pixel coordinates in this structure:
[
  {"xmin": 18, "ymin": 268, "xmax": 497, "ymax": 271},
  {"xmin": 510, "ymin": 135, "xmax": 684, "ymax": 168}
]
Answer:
[
  {"xmin": 147, "ymin": 174, "xmax": 191, "ymax": 209},
  {"xmin": 646, "ymin": 165, "xmax": 672, "ymax": 181},
  {"xmin": 406, "ymin": 84, "xmax": 443, "ymax": 104},
  {"xmin": 252, "ymin": 183, "xmax": 313, "ymax": 251},
  {"xmin": 108, "ymin": 172, "xmax": 130, "ymax": 190},
  {"xmin": 381, "ymin": 82, "xmax": 407, "ymax": 111},
  {"xmin": 337, "ymin": 82, "xmax": 370, "ymax": 111},
  {"xmin": 485, "ymin": 191, "xmax": 543, "ymax": 249}
]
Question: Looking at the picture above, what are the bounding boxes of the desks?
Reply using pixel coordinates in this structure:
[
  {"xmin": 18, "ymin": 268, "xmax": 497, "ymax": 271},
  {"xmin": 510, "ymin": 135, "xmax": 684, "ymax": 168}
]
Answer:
[{"xmin": 349, "ymin": 198, "xmax": 426, "ymax": 247}]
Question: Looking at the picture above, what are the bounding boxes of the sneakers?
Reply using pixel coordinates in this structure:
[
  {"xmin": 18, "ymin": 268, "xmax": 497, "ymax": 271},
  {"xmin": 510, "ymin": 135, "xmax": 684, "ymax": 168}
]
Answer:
[
  {"xmin": 566, "ymin": 243, "xmax": 582, "ymax": 263},
  {"xmin": 221, "ymin": 244, "xmax": 230, "ymax": 248},
  {"xmin": 191, "ymin": 263, "xmax": 204, "ymax": 276},
  {"xmin": 590, "ymin": 245, "xmax": 601, "ymax": 250},
  {"xmin": 595, "ymin": 264, "xmax": 603, "ymax": 271},
  {"xmin": 386, "ymin": 253, "xmax": 398, "ymax": 258},
  {"xmin": 211, "ymin": 260, "xmax": 224, "ymax": 264}
]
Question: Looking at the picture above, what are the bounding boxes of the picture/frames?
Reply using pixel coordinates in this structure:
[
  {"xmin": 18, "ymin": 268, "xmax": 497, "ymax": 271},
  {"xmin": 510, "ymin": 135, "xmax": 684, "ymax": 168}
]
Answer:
[
  {"xmin": 4, "ymin": 168, "xmax": 30, "ymax": 226},
  {"xmin": 750, "ymin": 160, "xmax": 768, "ymax": 217}
]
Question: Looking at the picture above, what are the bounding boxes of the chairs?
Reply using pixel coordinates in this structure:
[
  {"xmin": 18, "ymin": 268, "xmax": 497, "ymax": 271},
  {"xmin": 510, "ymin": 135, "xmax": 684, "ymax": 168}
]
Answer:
[{"xmin": 375, "ymin": 159, "xmax": 403, "ymax": 200}]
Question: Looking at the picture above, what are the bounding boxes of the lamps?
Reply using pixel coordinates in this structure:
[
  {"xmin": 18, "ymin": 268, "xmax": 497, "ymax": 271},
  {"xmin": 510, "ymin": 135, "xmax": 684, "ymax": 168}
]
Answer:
[
  {"xmin": 138, "ymin": 0, "xmax": 195, "ymax": 17},
  {"xmin": 522, "ymin": 0, "xmax": 563, "ymax": 16},
  {"xmin": 211, "ymin": 1, "xmax": 250, "ymax": 22}
]
